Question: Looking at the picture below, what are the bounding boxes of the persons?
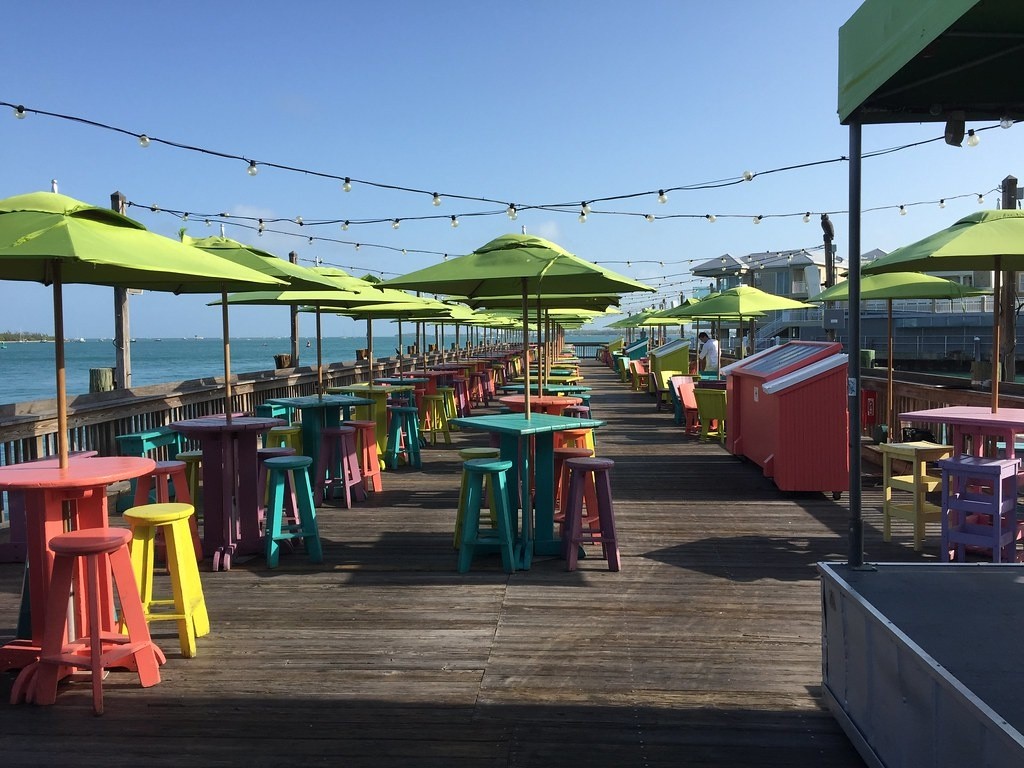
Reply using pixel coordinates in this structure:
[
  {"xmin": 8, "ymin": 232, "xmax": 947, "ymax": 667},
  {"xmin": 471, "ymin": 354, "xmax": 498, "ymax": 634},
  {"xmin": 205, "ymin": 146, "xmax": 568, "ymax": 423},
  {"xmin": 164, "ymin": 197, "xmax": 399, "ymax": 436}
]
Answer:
[{"xmin": 697, "ymin": 332, "xmax": 721, "ymax": 372}]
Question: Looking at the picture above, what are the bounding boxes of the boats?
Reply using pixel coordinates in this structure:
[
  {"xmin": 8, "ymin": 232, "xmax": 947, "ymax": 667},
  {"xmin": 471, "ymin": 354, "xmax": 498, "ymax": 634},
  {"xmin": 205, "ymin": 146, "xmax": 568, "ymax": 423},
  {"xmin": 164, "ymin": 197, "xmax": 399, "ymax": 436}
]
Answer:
[
  {"xmin": 73, "ymin": 337, "xmax": 86, "ymax": 343},
  {"xmin": 306, "ymin": 339, "xmax": 311, "ymax": 347},
  {"xmin": 0, "ymin": 341, "xmax": 7, "ymax": 349},
  {"xmin": 194, "ymin": 334, "xmax": 204, "ymax": 340},
  {"xmin": 130, "ymin": 339, "xmax": 137, "ymax": 342}
]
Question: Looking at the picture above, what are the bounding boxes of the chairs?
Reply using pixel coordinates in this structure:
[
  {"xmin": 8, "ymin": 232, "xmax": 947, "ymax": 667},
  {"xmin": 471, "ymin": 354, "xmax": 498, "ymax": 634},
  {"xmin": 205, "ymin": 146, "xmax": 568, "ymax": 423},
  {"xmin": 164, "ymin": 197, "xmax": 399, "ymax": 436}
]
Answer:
[{"xmin": 594, "ymin": 348, "xmax": 735, "ymax": 447}]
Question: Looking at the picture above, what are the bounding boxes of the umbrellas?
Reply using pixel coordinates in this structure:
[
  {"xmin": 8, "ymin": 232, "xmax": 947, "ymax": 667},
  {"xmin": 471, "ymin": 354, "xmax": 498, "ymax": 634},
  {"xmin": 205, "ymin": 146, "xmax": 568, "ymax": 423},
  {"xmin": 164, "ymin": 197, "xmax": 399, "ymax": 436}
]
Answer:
[
  {"xmin": 804, "ymin": 273, "xmax": 994, "ymax": 440},
  {"xmin": 206, "ymin": 224, "xmax": 820, "ymax": 478},
  {"xmin": 178, "ymin": 229, "xmax": 360, "ymax": 423},
  {"xmin": 0, "ymin": 179, "xmax": 290, "ymax": 469},
  {"xmin": 840, "ymin": 210, "xmax": 1024, "ymax": 458}
]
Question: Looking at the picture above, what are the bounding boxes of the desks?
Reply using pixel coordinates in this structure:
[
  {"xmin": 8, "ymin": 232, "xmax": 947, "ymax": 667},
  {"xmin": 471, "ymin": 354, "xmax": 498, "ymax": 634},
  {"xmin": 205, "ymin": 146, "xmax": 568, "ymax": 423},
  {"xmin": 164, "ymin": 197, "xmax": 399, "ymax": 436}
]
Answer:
[
  {"xmin": 0, "ymin": 456, "xmax": 157, "ymax": 681},
  {"xmin": 169, "ymin": 343, "xmax": 729, "ymax": 560},
  {"xmin": 898, "ymin": 406, "xmax": 1024, "ymax": 562}
]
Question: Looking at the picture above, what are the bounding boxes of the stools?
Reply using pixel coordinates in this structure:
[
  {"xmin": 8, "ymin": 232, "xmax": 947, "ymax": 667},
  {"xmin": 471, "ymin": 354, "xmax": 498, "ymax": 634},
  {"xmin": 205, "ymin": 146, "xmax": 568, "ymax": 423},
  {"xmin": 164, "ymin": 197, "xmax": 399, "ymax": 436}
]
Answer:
[
  {"xmin": 879, "ymin": 440, "xmax": 955, "ymax": 553},
  {"xmin": 938, "ymin": 453, "xmax": 1021, "ymax": 563},
  {"xmin": 33, "ymin": 344, "xmax": 621, "ymax": 706}
]
